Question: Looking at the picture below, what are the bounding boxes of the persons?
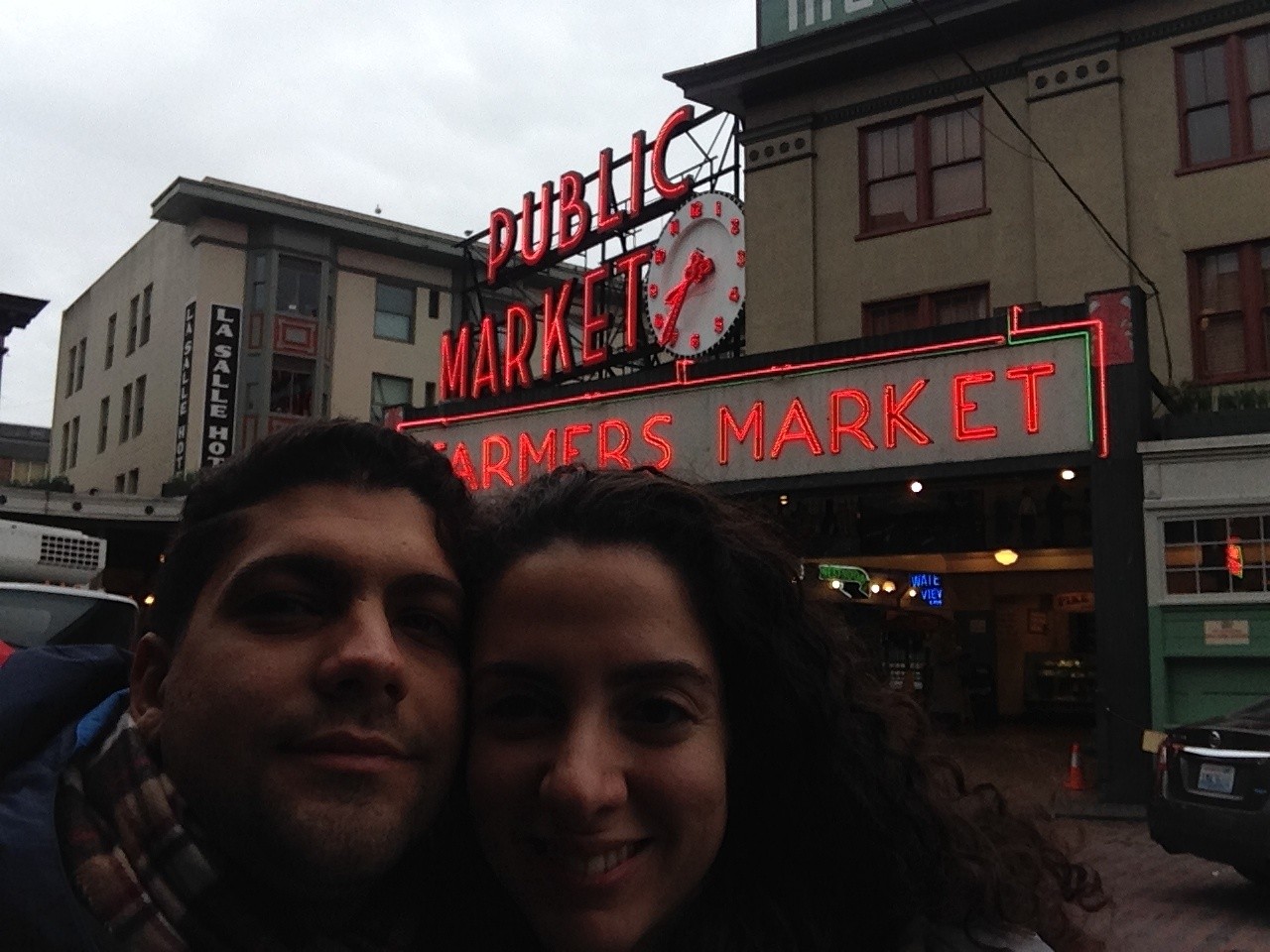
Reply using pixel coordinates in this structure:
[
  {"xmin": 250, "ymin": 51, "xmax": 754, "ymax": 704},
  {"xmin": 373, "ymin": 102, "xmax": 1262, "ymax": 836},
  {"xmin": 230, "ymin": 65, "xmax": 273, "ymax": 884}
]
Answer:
[{"xmin": 0, "ymin": 418, "xmax": 1105, "ymax": 952}]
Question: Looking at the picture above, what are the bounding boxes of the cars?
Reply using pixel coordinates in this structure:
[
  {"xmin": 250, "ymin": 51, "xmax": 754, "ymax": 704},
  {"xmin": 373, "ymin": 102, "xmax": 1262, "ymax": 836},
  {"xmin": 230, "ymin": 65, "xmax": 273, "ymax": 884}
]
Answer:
[{"xmin": 1146, "ymin": 697, "xmax": 1270, "ymax": 883}]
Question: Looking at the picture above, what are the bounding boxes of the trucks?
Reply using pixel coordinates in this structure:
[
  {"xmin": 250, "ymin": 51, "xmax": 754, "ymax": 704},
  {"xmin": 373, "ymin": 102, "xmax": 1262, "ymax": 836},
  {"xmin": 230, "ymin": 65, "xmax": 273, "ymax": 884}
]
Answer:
[{"xmin": 0, "ymin": 519, "xmax": 139, "ymax": 666}]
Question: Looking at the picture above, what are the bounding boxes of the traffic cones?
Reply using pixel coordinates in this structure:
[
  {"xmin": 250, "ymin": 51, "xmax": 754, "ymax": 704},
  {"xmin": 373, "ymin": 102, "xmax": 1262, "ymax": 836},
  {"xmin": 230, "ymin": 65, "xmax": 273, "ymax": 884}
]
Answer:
[{"xmin": 1064, "ymin": 743, "xmax": 1087, "ymax": 790}]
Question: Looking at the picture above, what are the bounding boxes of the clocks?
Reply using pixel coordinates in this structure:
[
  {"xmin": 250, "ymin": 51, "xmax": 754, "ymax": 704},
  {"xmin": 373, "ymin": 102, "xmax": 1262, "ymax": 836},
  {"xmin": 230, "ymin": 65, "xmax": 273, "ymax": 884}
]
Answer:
[{"xmin": 645, "ymin": 190, "xmax": 748, "ymax": 358}]
